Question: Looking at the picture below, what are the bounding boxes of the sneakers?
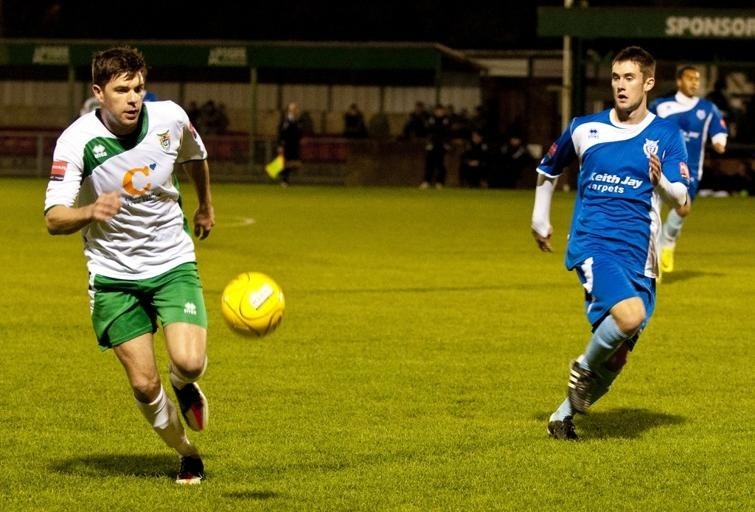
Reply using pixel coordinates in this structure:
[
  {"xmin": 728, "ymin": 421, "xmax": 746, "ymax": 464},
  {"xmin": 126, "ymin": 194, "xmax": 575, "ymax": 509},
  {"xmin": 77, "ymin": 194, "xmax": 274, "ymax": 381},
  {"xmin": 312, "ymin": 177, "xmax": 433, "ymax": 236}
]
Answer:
[
  {"xmin": 170, "ymin": 380, "xmax": 208, "ymax": 433},
  {"xmin": 546, "ymin": 419, "xmax": 578, "ymax": 442},
  {"xmin": 567, "ymin": 360, "xmax": 592, "ymax": 416},
  {"xmin": 660, "ymin": 247, "xmax": 674, "ymax": 273},
  {"xmin": 176, "ymin": 454, "xmax": 205, "ymax": 486}
]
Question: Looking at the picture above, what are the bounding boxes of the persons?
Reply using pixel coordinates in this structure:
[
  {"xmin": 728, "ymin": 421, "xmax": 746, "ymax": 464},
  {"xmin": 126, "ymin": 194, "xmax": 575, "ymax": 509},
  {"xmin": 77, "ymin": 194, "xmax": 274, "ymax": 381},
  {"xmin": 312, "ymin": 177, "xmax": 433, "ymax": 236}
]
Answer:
[
  {"xmin": 278, "ymin": 104, "xmax": 304, "ymax": 186},
  {"xmin": 186, "ymin": 101, "xmax": 199, "ymax": 134},
  {"xmin": 407, "ymin": 98, "xmax": 528, "ymax": 189},
  {"xmin": 198, "ymin": 100, "xmax": 224, "ymax": 133},
  {"xmin": 43, "ymin": 46, "xmax": 218, "ymax": 486},
  {"xmin": 529, "ymin": 48, "xmax": 691, "ymax": 440},
  {"xmin": 653, "ymin": 64, "xmax": 731, "ymax": 280},
  {"xmin": 344, "ymin": 102, "xmax": 367, "ymax": 137}
]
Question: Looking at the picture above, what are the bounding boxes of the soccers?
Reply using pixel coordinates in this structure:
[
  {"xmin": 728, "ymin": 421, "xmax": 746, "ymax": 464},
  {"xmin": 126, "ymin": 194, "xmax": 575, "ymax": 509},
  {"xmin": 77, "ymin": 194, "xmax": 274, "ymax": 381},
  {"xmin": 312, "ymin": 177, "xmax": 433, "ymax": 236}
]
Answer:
[{"xmin": 222, "ymin": 271, "xmax": 283, "ymax": 338}]
